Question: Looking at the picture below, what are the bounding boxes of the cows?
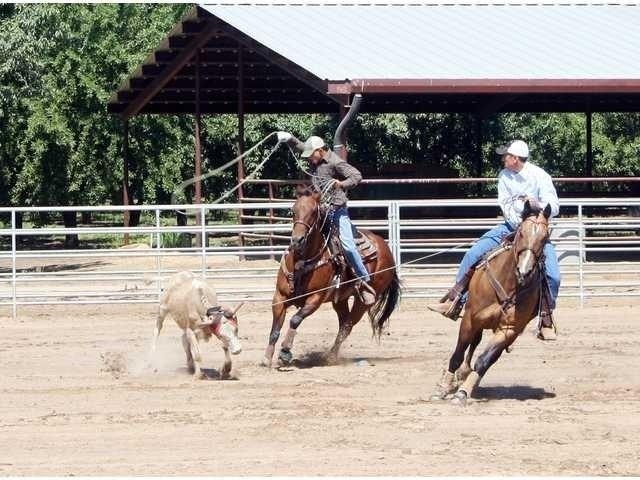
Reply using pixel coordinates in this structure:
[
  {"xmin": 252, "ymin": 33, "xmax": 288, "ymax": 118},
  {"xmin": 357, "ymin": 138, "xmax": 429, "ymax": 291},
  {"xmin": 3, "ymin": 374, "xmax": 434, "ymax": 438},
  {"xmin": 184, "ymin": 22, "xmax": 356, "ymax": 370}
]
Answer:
[{"xmin": 153, "ymin": 272, "xmax": 245, "ymax": 381}]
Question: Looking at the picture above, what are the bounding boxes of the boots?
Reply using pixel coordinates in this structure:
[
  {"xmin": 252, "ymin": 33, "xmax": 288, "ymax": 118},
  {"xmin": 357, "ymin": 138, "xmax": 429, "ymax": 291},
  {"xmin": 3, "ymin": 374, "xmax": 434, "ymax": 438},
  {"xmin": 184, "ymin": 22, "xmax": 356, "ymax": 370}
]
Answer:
[
  {"xmin": 541, "ymin": 315, "xmax": 556, "ymax": 340},
  {"xmin": 358, "ymin": 283, "xmax": 375, "ymax": 305},
  {"xmin": 428, "ymin": 301, "xmax": 461, "ymax": 320}
]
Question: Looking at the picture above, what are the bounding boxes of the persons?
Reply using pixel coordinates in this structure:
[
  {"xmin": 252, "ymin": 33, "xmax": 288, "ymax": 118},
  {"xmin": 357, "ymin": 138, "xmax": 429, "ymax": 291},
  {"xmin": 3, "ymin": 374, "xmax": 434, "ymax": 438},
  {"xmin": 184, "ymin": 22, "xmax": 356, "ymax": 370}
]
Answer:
[
  {"xmin": 426, "ymin": 139, "xmax": 561, "ymax": 341},
  {"xmin": 276, "ymin": 130, "xmax": 376, "ymax": 306}
]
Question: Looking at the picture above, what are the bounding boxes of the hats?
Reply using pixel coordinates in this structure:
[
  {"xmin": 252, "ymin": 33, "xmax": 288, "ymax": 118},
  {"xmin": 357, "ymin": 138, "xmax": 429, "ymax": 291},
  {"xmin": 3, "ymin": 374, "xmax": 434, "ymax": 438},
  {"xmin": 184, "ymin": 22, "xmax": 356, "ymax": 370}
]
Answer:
[
  {"xmin": 496, "ymin": 139, "xmax": 529, "ymax": 158},
  {"xmin": 301, "ymin": 135, "xmax": 324, "ymax": 157}
]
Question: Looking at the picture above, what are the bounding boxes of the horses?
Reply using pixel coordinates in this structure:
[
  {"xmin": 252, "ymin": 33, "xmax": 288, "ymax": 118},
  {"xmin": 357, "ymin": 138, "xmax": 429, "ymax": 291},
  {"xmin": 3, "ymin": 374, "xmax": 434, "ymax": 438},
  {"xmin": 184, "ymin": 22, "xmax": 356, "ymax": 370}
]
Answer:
[
  {"xmin": 260, "ymin": 186, "xmax": 401, "ymax": 370},
  {"xmin": 431, "ymin": 199, "xmax": 552, "ymax": 403}
]
